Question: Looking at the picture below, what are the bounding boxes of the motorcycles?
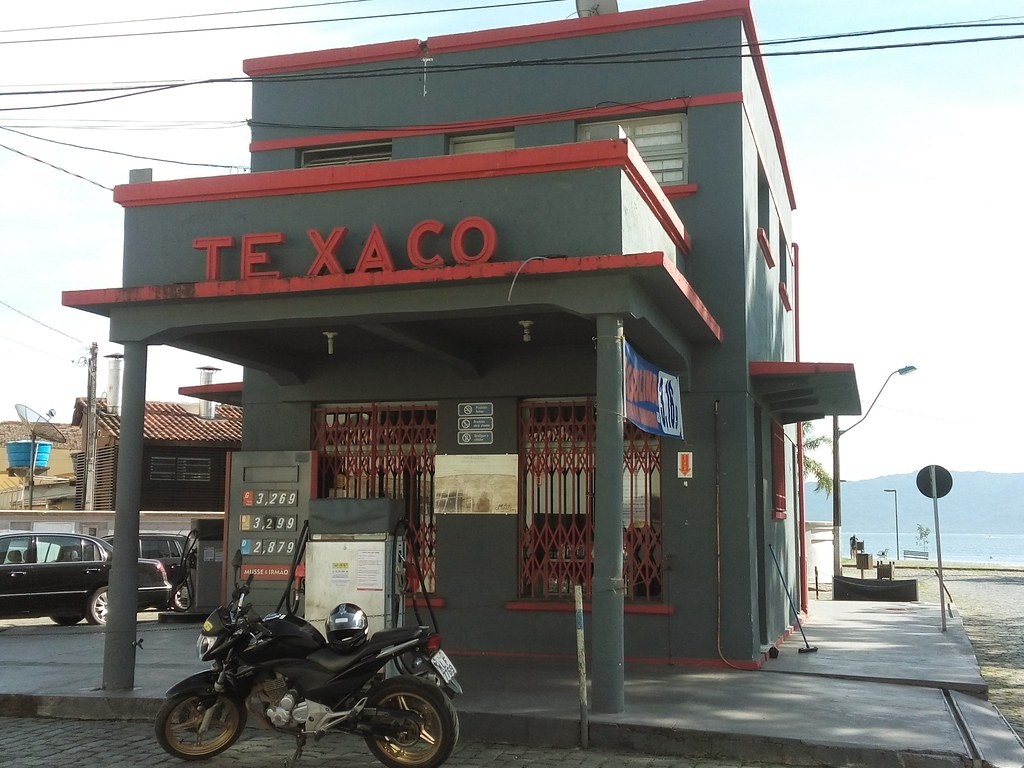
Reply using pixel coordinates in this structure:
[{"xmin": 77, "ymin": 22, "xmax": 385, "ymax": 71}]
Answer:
[{"xmin": 153, "ymin": 548, "xmax": 462, "ymax": 768}]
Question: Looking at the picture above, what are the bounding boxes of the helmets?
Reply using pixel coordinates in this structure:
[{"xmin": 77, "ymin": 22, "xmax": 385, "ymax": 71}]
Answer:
[{"xmin": 325, "ymin": 602, "xmax": 368, "ymax": 655}]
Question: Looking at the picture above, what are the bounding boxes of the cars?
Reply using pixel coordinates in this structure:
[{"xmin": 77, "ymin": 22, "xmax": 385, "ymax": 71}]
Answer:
[{"xmin": 0, "ymin": 532, "xmax": 171, "ymax": 624}]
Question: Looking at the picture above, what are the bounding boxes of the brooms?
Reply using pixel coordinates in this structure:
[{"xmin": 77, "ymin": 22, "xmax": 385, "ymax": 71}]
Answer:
[{"xmin": 769, "ymin": 544, "xmax": 818, "ymax": 653}]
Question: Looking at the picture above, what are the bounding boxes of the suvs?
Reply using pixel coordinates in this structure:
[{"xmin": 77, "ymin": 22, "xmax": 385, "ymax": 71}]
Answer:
[{"xmin": 98, "ymin": 532, "xmax": 195, "ymax": 611}]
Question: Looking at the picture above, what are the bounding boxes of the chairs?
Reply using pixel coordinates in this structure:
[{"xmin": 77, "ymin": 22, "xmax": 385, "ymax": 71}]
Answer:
[
  {"xmin": 23, "ymin": 550, "xmax": 39, "ymax": 564},
  {"xmin": 5, "ymin": 550, "xmax": 22, "ymax": 564},
  {"xmin": 60, "ymin": 550, "xmax": 79, "ymax": 561}
]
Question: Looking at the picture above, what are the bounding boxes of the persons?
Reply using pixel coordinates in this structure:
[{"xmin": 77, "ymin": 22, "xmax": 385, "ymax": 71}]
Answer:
[{"xmin": 850, "ymin": 533, "xmax": 859, "ymax": 560}]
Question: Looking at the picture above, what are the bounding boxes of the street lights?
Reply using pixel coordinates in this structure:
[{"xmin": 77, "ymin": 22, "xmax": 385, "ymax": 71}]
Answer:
[
  {"xmin": 884, "ymin": 490, "xmax": 899, "ymax": 560},
  {"xmin": 832, "ymin": 365, "xmax": 917, "ymax": 576}
]
